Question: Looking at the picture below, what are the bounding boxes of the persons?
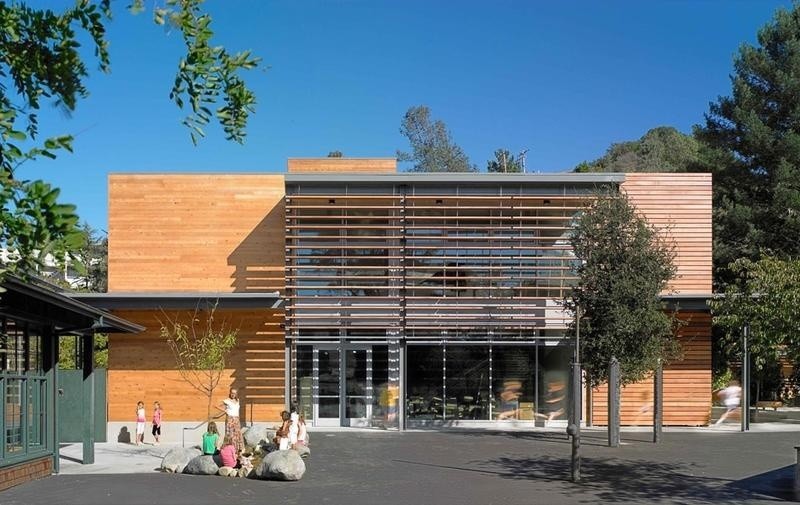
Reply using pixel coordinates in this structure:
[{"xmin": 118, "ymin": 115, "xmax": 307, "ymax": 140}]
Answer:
[
  {"xmin": 496, "ymin": 382, "xmax": 522, "ymax": 419},
  {"xmin": 715, "ymin": 380, "xmax": 743, "ymax": 428},
  {"xmin": 546, "ymin": 383, "xmax": 566, "ymax": 425},
  {"xmin": 381, "ymin": 383, "xmax": 398, "ymax": 420},
  {"xmin": 203, "ymin": 387, "xmax": 307, "ymax": 469},
  {"xmin": 136, "ymin": 401, "xmax": 146, "ymax": 445},
  {"xmin": 152, "ymin": 399, "xmax": 161, "ymax": 443}
]
